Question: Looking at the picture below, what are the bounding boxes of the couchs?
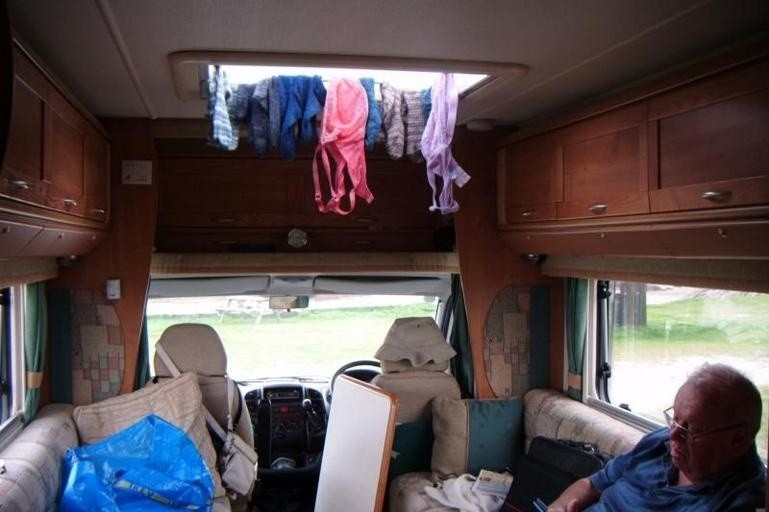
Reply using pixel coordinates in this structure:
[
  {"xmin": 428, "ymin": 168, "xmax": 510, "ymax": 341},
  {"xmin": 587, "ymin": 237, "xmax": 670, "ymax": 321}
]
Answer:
[
  {"xmin": 388, "ymin": 389, "xmax": 646, "ymax": 511},
  {"xmin": 1, "ymin": 403, "xmax": 231, "ymax": 512}
]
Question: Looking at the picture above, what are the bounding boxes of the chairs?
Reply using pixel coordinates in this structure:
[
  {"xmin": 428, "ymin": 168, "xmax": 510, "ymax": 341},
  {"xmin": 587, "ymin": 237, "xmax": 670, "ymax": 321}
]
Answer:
[
  {"xmin": 145, "ymin": 323, "xmax": 251, "ymax": 451},
  {"xmin": 372, "ymin": 318, "xmax": 462, "ymax": 424}
]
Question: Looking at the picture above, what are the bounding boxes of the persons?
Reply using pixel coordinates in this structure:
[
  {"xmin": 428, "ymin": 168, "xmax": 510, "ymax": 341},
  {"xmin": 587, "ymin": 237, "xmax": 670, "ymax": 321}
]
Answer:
[{"xmin": 539, "ymin": 361, "xmax": 768, "ymax": 512}]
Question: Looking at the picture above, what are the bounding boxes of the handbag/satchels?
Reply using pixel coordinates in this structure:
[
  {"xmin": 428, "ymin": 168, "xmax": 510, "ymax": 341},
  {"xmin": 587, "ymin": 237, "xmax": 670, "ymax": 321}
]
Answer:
[
  {"xmin": 220, "ymin": 429, "xmax": 258, "ymax": 497},
  {"xmin": 57, "ymin": 413, "xmax": 214, "ymax": 512},
  {"xmin": 511, "ymin": 436, "xmax": 607, "ymax": 509}
]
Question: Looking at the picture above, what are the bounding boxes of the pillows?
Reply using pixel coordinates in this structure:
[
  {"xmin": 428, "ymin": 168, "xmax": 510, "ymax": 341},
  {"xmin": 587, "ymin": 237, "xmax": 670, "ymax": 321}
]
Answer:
[
  {"xmin": 73, "ymin": 376, "xmax": 226, "ymax": 498},
  {"xmin": 431, "ymin": 397, "xmax": 523, "ymax": 481}
]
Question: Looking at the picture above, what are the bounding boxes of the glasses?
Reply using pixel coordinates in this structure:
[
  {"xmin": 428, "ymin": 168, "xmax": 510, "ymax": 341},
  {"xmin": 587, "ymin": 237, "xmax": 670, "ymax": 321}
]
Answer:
[{"xmin": 663, "ymin": 405, "xmax": 750, "ymax": 441}]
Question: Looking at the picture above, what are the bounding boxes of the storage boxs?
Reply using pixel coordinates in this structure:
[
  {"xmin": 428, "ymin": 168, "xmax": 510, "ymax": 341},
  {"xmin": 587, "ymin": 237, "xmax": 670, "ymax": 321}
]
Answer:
[
  {"xmin": 496, "ymin": 129, "xmax": 560, "ymax": 232},
  {"xmin": 561, "ymin": 101, "xmax": 649, "ymax": 232},
  {"xmin": 650, "ymin": 61, "xmax": 768, "ymax": 233}
]
